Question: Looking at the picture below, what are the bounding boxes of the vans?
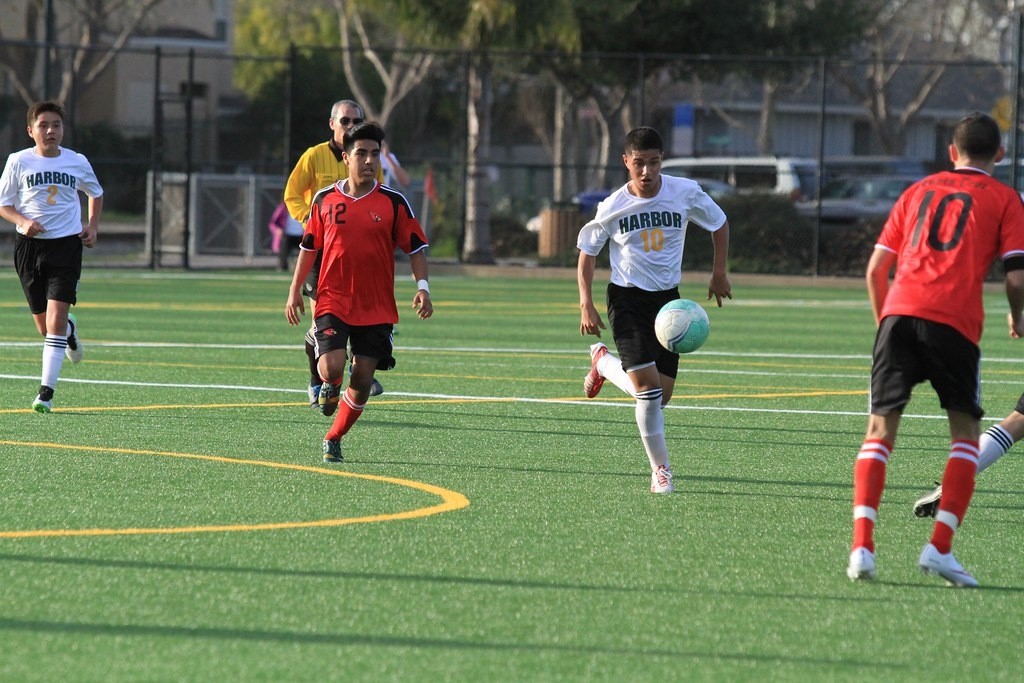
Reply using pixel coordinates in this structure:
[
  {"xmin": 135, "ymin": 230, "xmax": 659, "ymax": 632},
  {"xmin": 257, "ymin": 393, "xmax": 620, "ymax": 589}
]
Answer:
[{"xmin": 656, "ymin": 152, "xmax": 830, "ymax": 208}]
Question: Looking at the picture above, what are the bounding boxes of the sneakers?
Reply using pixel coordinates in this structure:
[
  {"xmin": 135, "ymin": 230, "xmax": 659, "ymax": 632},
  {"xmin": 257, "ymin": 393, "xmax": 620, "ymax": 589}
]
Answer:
[
  {"xmin": 322, "ymin": 432, "xmax": 343, "ymax": 465},
  {"xmin": 65, "ymin": 312, "xmax": 83, "ymax": 363},
  {"xmin": 318, "ymin": 380, "xmax": 344, "ymax": 416},
  {"xmin": 307, "ymin": 382, "xmax": 322, "ymax": 409},
  {"xmin": 584, "ymin": 342, "xmax": 608, "ymax": 398},
  {"xmin": 348, "ymin": 363, "xmax": 384, "ymax": 396},
  {"xmin": 651, "ymin": 465, "xmax": 674, "ymax": 494},
  {"xmin": 913, "ymin": 481, "xmax": 944, "ymax": 518},
  {"xmin": 920, "ymin": 542, "xmax": 980, "ymax": 588},
  {"xmin": 846, "ymin": 546, "xmax": 875, "ymax": 582},
  {"xmin": 31, "ymin": 388, "xmax": 53, "ymax": 413}
]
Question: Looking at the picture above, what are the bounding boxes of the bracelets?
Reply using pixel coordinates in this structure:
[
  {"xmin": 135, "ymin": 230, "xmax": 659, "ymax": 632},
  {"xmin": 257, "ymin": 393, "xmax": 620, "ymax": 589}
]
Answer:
[{"xmin": 417, "ymin": 279, "xmax": 430, "ymax": 294}]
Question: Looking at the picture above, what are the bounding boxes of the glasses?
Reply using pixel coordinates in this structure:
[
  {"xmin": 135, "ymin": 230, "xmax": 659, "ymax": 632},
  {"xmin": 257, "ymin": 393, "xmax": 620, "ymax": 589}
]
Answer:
[{"xmin": 332, "ymin": 116, "xmax": 362, "ymax": 126}]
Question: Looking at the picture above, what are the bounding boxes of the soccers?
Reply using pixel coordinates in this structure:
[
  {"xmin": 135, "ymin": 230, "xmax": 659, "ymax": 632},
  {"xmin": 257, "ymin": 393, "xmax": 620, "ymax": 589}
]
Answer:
[{"xmin": 653, "ymin": 298, "xmax": 711, "ymax": 354}]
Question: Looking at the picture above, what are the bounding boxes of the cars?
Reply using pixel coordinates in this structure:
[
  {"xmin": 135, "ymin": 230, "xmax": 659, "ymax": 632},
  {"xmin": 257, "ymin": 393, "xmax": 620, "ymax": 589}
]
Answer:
[{"xmin": 792, "ymin": 173, "xmax": 932, "ymax": 223}]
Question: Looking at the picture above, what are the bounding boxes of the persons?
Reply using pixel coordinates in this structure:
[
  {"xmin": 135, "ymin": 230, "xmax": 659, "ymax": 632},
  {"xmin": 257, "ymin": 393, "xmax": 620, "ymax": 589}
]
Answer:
[
  {"xmin": 272, "ymin": 100, "xmax": 434, "ymax": 461},
  {"xmin": 844, "ymin": 113, "xmax": 1024, "ymax": 589},
  {"xmin": 575, "ymin": 127, "xmax": 734, "ymax": 496},
  {"xmin": 0, "ymin": 103, "xmax": 101, "ymax": 413}
]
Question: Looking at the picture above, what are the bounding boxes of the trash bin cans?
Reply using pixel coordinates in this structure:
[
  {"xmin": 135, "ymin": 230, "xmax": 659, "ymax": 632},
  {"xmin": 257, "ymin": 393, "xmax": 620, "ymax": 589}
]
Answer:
[{"xmin": 537, "ymin": 197, "xmax": 584, "ymax": 266}]
